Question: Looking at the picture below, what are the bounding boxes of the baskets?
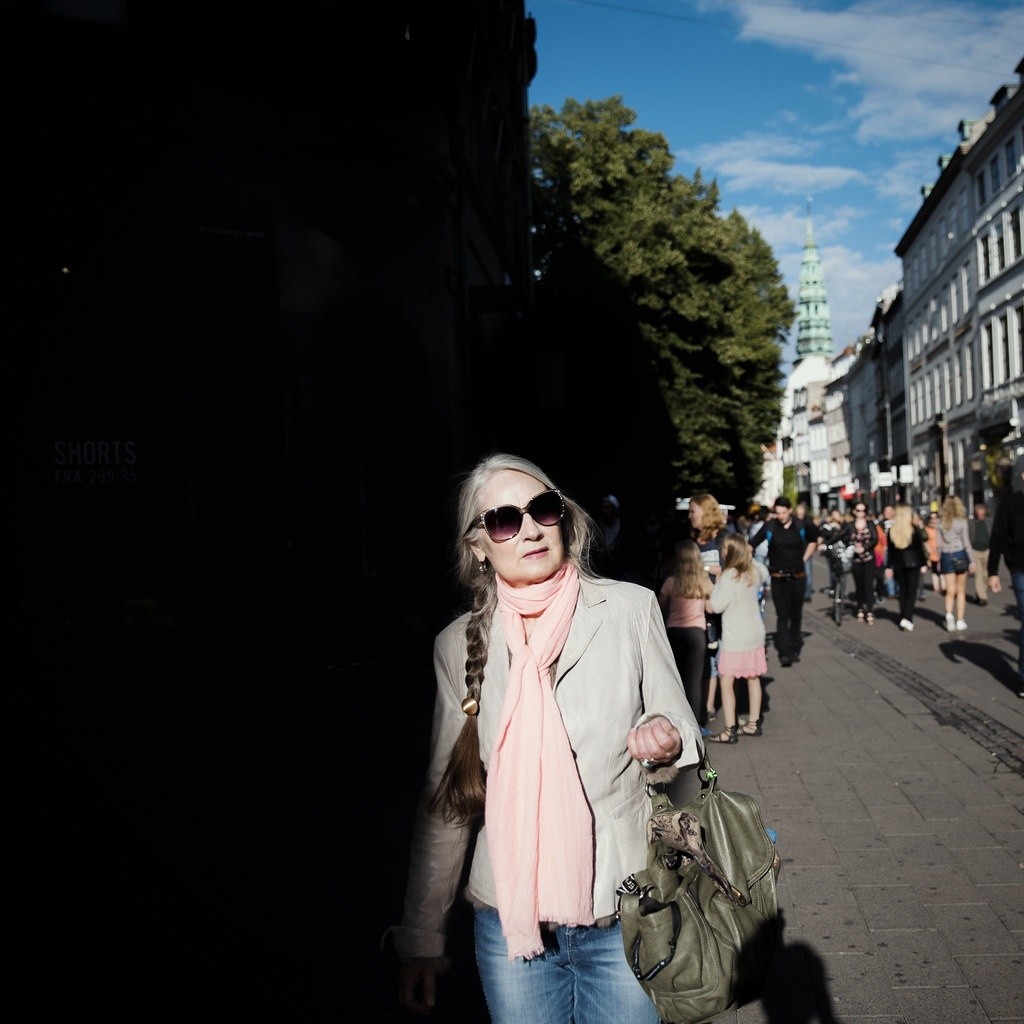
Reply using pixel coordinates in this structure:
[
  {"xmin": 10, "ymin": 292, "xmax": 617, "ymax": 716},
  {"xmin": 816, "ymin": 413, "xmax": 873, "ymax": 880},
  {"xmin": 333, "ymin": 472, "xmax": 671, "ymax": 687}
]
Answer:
[{"xmin": 830, "ymin": 553, "xmax": 853, "ymax": 573}]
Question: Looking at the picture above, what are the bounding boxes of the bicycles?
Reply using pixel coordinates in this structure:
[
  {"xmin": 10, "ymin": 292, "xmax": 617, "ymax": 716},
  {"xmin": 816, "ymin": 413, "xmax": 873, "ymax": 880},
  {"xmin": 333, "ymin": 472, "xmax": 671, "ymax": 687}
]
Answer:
[{"xmin": 820, "ymin": 540, "xmax": 853, "ymax": 621}]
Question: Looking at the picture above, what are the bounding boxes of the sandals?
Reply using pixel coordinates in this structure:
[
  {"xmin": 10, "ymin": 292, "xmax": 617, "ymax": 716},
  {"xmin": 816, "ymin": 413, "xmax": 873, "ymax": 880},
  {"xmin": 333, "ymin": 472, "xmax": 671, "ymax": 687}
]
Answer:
[
  {"xmin": 709, "ymin": 726, "xmax": 738, "ymax": 743},
  {"xmin": 737, "ymin": 719, "xmax": 762, "ymax": 736}
]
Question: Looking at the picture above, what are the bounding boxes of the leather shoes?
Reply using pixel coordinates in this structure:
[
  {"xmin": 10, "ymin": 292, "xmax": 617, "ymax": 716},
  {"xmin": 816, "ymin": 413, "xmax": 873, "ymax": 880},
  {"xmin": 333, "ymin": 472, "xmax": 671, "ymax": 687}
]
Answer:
[{"xmin": 975, "ymin": 598, "xmax": 988, "ymax": 606}]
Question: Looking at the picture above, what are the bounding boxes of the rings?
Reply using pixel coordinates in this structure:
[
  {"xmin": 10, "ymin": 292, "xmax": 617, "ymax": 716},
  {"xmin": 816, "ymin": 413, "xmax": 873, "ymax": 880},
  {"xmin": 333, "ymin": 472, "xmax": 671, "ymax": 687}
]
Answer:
[{"xmin": 641, "ymin": 756, "xmax": 655, "ymax": 769}]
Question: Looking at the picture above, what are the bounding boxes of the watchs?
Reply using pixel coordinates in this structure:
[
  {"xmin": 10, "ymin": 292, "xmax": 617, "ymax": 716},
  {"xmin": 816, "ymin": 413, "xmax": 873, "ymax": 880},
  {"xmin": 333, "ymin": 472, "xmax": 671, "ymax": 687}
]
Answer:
[{"xmin": 704, "ymin": 564, "xmax": 710, "ymax": 572}]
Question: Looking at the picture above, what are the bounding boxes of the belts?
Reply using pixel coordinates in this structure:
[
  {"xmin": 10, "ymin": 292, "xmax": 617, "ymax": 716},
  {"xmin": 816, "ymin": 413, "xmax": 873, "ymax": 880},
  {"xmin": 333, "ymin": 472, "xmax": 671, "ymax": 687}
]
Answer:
[{"xmin": 771, "ymin": 572, "xmax": 806, "ymax": 578}]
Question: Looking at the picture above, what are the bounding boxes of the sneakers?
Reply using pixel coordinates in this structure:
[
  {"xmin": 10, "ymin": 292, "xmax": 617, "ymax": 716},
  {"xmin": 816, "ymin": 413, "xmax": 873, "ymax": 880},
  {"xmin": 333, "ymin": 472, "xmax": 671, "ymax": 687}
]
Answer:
[
  {"xmin": 899, "ymin": 618, "xmax": 913, "ymax": 631},
  {"xmin": 942, "ymin": 614, "xmax": 956, "ymax": 631},
  {"xmin": 956, "ymin": 619, "xmax": 968, "ymax": 630}
]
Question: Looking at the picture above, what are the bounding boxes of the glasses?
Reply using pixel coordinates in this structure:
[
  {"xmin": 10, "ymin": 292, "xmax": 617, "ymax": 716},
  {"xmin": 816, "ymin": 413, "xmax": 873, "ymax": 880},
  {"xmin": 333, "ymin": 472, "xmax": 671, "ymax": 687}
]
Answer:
[
  {"xmin": 467, "ymin": 489, "xmax": 566, "ymax": 544},
  {"xmin": 855, "ymin": 508, "xmax": 866, "ymax": 512}
]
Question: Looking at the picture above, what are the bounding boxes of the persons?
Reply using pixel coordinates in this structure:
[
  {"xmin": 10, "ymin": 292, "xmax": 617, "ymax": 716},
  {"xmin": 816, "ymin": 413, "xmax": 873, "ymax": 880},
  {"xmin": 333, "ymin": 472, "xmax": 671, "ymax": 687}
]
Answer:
[
  {"xmin": 397, "ymin": 453, "xmax": 788, "ymax": 1024},
  {"xmin": 594, "ymin": 453, "xmax": 1024, "ymax": 745}
]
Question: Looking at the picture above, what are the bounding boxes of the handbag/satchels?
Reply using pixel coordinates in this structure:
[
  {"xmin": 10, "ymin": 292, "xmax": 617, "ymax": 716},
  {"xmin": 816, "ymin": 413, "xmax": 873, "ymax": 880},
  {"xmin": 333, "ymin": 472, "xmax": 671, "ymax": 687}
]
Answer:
[
  {"xmin": 617, "ymin": 749, "xmax": 781, "ymax": 1023},
  {"xmin": 952, "ymin": 558, "xmax": 968, "ymax": 575}
]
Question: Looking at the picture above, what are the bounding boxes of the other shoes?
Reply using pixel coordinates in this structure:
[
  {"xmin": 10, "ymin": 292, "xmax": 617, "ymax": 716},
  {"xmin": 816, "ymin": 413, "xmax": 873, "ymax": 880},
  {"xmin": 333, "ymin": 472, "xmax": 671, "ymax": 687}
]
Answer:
[
  {"xmin": 781, "ymin": 660, "xmax": 791, "ymax": 667},
  {"xmin": 867, "ymin": 612, "xmax": 875, "ymax": 626},
  {"xmin": 857, "ymin": 609, "xmax": 864, "ymax": 622}
]
